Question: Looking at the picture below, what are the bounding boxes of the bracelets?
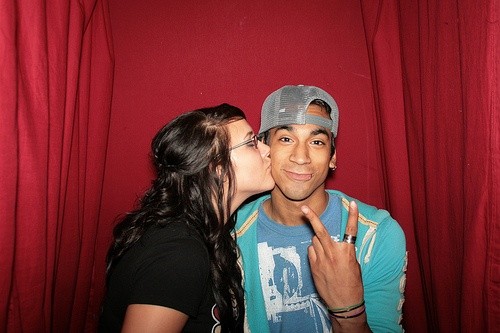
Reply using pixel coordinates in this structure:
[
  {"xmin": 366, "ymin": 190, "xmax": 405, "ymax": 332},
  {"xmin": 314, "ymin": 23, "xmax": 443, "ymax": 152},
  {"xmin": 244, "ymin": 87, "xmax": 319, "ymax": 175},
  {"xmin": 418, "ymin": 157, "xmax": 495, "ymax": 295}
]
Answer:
[{"xmin": 328, "ymin": 301, "xmax": 366, "ymax": 319}]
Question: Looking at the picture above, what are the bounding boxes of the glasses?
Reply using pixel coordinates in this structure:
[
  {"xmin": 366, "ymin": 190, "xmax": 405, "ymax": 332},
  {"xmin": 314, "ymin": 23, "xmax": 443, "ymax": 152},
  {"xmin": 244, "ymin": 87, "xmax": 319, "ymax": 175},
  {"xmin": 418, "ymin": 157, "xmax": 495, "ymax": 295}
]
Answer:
[{"xmin": 228, "ymin": 132, "xmax": 266, "ymax": 150}]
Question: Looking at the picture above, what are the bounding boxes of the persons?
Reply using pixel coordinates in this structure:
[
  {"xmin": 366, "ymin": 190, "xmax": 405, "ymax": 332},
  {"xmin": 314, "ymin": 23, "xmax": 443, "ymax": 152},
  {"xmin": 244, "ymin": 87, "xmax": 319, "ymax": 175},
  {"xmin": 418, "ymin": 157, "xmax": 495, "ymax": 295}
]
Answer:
[
  {"xmin": 100, "ymin": 103, "xmax": 275, "ymax": 333},
  {"xmin": 228, "ymin": 85, "xmax": 409, "ymax": 333}
]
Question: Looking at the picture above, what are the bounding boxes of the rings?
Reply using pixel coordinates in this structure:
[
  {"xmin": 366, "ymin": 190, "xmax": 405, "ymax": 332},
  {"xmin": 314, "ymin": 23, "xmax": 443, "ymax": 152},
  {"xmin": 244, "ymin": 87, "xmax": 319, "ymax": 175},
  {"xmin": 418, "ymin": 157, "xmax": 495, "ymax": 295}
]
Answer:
[{"xmin": 343, "ymin": 233, "xmax": 357, "ymax": 244}]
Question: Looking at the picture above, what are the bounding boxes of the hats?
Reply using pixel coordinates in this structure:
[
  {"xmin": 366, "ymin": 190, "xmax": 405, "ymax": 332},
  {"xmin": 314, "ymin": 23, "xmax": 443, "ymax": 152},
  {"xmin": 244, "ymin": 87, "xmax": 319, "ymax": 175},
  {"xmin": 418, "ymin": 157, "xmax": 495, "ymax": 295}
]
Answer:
[{"xmin": 257, "ymin": 84, "xmax": 340, "ymax": 137}]
